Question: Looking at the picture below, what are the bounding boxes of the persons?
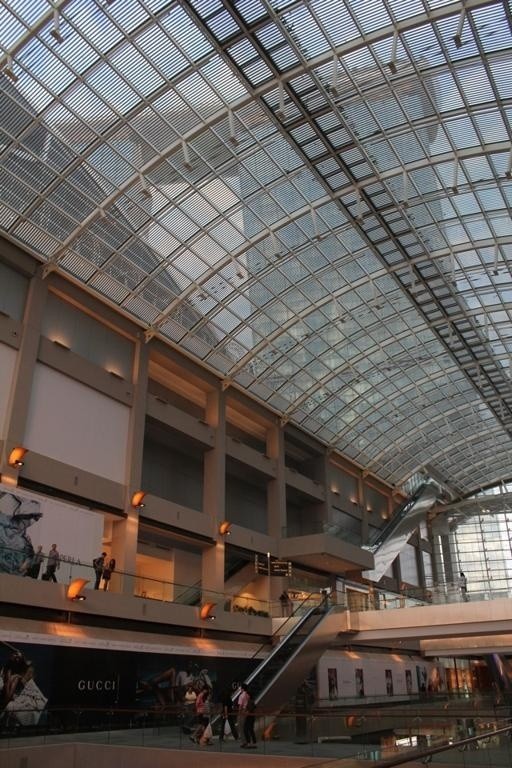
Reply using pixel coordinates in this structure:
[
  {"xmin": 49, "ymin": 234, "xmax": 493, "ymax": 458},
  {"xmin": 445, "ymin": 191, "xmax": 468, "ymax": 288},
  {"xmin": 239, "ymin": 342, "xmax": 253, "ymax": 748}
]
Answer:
[
  {"xmin": 43, "ymin": 543, "xmax": 61, "ymax": 583},
  {"xmin": 103, "ymin": 558, "xmax": 116, "ymax": 591},
  {"xmin": 183, "ymin": 680, "xmax": 258, "ymax": 748},
  {"xmin": 280, "ymin": 590, "xmax": 289, "ymax": 617},
  {"xmin": 94, "ymin": 551, "xmax": 108, "ymax": 590},
  {"xmin": 29, "ymin": 544, "xmax": 46, "ymax": 578},
  {"xmin": 459, "ymin": 572, "xmax": 467, "ymax": 602},
  {"xmin": 140, "ymin": 660, "xmax": 211, "ymax": 706},
  {"xmin": 6, "ymin": 648, "xmax": 32, "ymax": 701}
]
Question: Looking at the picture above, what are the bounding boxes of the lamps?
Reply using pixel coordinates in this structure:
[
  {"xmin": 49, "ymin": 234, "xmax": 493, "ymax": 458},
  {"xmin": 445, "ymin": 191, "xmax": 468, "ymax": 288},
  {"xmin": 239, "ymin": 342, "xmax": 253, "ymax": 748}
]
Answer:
[
  {"xmin": 66, "ymin": 574, "xmax": 87, "ymax": 604},
  {"xmin": 7, "ymin": 444, "xmax": 28, "ymax": 470},
  {"xmin": 131, "ymin": 487, "xmax": 149, "ymax": 511},
  {"xmin": 200, "ymin": 598, "xmax": 219, "ymax": 624},
  {"xmin": 219, "ymin": 518, "xmax": 233, "ymax": 537}
]
